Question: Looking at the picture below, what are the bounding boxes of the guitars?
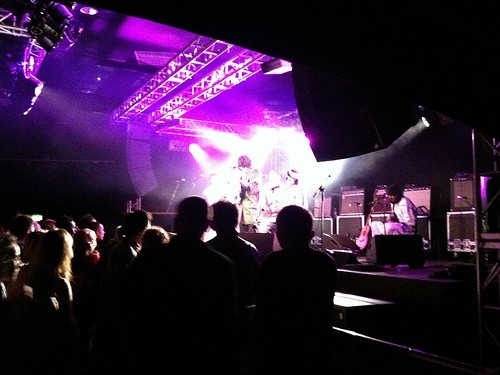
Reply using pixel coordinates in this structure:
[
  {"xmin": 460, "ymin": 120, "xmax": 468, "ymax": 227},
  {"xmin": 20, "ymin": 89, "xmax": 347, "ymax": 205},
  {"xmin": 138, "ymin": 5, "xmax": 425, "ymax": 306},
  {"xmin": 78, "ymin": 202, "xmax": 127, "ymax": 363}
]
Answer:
[{"xmin": 356, "ymin": 214, "xmax": 372, "ymax": 251}]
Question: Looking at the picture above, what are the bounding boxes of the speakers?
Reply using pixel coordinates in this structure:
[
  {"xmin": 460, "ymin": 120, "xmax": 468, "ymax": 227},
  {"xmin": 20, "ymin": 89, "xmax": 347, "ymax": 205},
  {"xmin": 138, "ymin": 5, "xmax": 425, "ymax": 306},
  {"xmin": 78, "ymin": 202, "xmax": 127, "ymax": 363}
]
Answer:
[
  {"xmin": 321, "ymin": 234, "xmax": 363, "ymax": 264},
  {"xmin": 340, "ymin": 194, "xmax": 364, "ymax": 215},
  {"xmin": 366, "ymin": 234, "xmax": 426, "ymax": 269},
  {"xmin": 314, "ymin": 195, "xmax": 339, "ymax": 218},
  {"xmin": 238, "ymin": 232, "xmax": 282, "ymax": 258},
  {"xmin": 373, "ymin": 190, "xmax": 394, "ymax": 213},
  {"xmin": 450, "ymin": 180, "xmax": 476, "ymax": 210},
  {"xmin": 403, "ymin": 188, "xmax": 431, "ymax": 217}
]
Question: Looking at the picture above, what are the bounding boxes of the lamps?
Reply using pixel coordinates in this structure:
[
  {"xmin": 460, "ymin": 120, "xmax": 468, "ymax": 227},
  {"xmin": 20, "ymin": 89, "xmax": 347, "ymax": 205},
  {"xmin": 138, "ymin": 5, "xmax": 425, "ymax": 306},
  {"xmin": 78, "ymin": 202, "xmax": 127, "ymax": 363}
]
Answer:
[
  {"xmin": 26, "ymin": 0, "xmax": 76, "ymax": 53},
  {"xmin": 418, "ymin": 104, "xmax": 433, "ymax": 128},
  {"xmin": 260, "ymin": 58, "xmax": 293, "ymax": 76}
]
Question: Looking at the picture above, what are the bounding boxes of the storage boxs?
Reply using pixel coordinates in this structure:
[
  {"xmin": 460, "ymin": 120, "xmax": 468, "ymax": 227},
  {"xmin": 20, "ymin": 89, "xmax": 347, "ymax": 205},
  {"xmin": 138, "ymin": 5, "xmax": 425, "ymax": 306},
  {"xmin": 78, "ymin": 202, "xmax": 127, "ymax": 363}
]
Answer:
[
  {"xmin": 335, "ymin": 215, "xmax": 365, "ymax": 237},
  {"xmin": 445, "ymin": 211, "xmax": 477, "ymax": 253},
  {"xmin": 313, "ymin": 218, "xmax": 333, "ymax": 238},
  {"xmin": 416, "ymin": 216, "xmax": 432, "ymax": 240},
  {"xmin": 449, "ymin": 176, "xmax": 475, "ymax": 211},
  {"xmin": 338, "ymin": 193, "xmax": 365, "ymax": 216}
]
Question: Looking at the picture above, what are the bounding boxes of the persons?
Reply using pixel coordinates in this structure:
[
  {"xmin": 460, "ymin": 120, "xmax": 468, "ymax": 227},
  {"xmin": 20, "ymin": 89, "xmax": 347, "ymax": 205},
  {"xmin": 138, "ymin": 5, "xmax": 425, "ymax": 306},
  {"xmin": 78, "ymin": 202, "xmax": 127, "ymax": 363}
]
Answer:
[
  {"xmin": 203, "ymin": 154, "xmax": 304, "ymax": 233},
  {"xmin": 371, "ymin": 184, "xmax": 418, "ymax": 234},
  {"xmin": 0, "ymin": 196, "xmax": 337, "ymax": 375}
]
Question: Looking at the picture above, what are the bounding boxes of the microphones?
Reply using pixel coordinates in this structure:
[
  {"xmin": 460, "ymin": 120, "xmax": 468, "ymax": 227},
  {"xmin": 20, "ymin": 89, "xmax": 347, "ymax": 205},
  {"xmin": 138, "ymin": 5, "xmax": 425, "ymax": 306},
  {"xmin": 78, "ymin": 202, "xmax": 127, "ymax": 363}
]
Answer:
[
  {"xmin": 457, "ymin": 195, "xmax": 463, "ymax": 199},
  {"xmin": 177, "ymin": 178, "xmax": 185, "ymax": 183}
]
